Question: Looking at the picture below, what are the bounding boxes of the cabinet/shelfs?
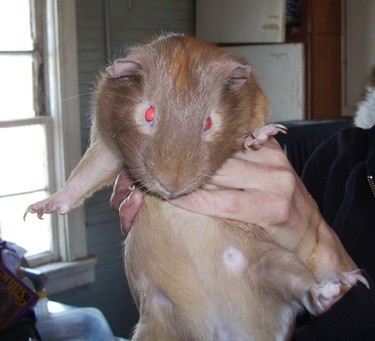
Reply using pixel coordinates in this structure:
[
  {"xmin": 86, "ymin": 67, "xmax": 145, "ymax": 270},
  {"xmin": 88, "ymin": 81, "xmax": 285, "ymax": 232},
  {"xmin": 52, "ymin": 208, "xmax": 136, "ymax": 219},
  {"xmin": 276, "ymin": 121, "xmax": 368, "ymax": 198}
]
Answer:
[{"xmin": 191, "ymin": 0, "xmax": 342, "ymax": 127}]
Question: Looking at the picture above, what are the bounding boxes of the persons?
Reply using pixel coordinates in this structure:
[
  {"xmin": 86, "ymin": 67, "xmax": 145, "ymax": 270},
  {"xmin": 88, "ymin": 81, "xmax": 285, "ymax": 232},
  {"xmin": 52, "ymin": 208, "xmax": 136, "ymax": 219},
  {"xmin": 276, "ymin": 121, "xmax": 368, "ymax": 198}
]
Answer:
[{"xmin": 110, "ymin": 126, "xmax": 375, "ymax": 341}]
